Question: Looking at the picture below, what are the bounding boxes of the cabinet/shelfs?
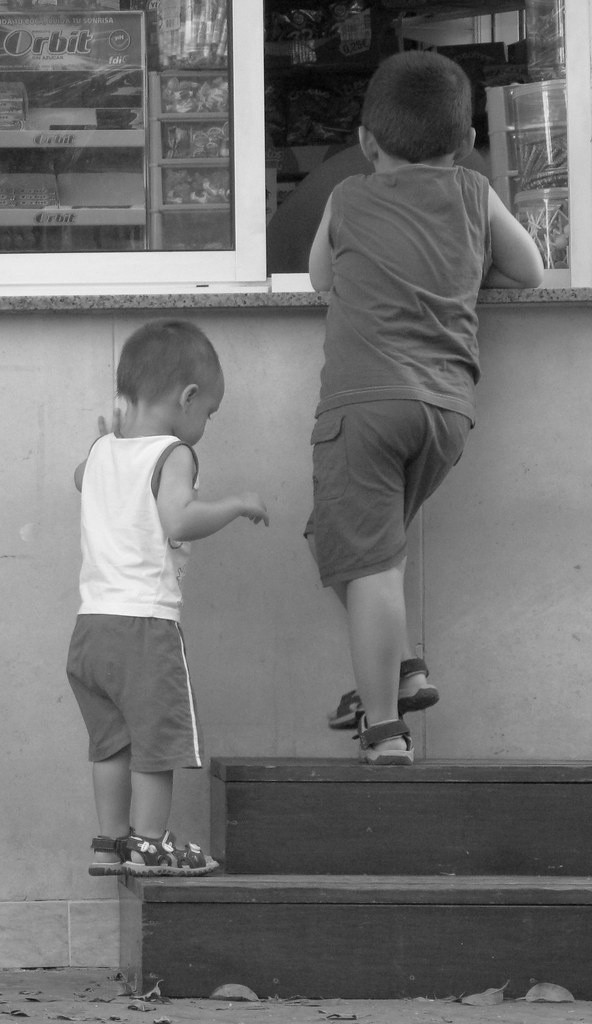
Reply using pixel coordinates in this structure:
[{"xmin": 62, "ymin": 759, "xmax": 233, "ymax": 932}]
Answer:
[
  {"xmin": 0, "ymin": 12, "xmax": 147, "ymax": 253},
  {"xmin": 148, "ymin": 72, "xmax": 235, "ymax": 252},
  {"xmin": 486, "ymin": 78, "xmax": 568, "ymax": 271}
]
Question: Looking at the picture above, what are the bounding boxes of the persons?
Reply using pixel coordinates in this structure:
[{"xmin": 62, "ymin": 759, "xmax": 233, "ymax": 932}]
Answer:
[
  {"xmin": 301, "ymin": 48, "xmax": 545, "ymax": 765},
  {"xmin": 265, "ymin": 46, "xmax": 535, "ymax": 272},
  {"xmin": 66, "ymin": 319, "xmax": 270, "ymax": 877}
]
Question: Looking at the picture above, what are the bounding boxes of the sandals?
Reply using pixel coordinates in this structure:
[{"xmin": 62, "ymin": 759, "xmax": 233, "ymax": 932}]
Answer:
[
  {"xmin": 88, "ymin": 836, "xmax": 130, "ymax": 876},
  {"xmin": 123, "ymin": 830, "xmax": 219, "ymax": 878},
  {"xmin": 329, "ymin": 658, "xmax": 440, "ymax": 729},
  {"xmin": 351, "ymin": 711, "xmax": 415, "ymax": 765}
]
